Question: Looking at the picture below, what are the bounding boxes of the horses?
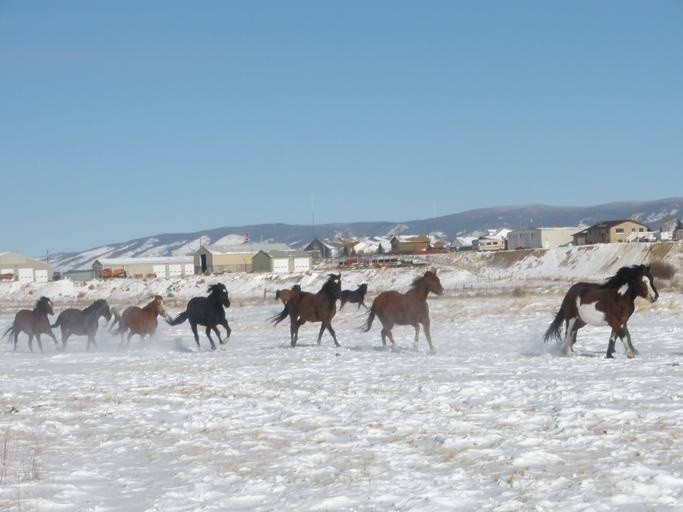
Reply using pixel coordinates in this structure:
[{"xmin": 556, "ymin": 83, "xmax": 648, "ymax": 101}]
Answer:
[
  {"xmin": 543, "ymin": 263, "xmax": 660, "ymax": 359},
  {"xmin": 274, "ymin": 284, "xmax": 302, "ymax": 308},
  {"xmin": 102, "ymin": 305, "xmax": 130, "ymax": 336},
  {"xmin": 337, "ymin": 283, "xmax": 369, "ymax": 311},
  {"xmin": 355, "ymin": 269, "xmax": 444, "ymax": 352},
  {"xmin": 3, "ymin": 297, "xmax": 61, "ymax": 352},
  {"xmin": 50, "ymin": 298, "xmax": 113, "ymax": 352},
  {"xmin": 263, "ymin": 272, "xmax": 343, "ymax": 347},
  {"xmin": 166, "ymin": 282, "xmax": 232, "ymax": 350},
  {"xmin": 112, "ymin": 295, "xmax": 167, "ymax": 345}
]
